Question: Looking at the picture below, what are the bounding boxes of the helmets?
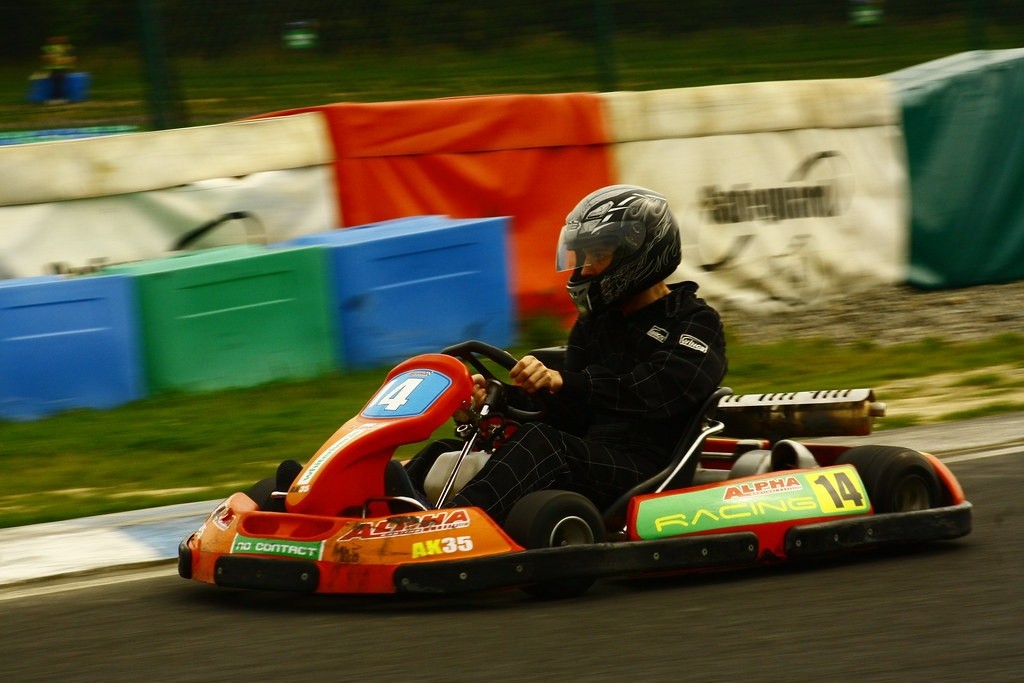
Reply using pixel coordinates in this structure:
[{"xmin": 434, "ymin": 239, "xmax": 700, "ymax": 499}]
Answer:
[{"xmin": 555, "ymin": 185, "xmax": 682, "ymax": 316}]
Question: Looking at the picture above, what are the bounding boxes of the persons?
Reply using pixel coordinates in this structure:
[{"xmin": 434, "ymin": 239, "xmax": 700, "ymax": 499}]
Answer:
[{"xmin": 275, "ymin": 184, "xmax": 728, "ymax": 526}]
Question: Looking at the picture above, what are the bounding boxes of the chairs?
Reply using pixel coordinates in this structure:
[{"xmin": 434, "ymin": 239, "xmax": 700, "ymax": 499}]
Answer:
[{"xmin": 600, "ymin": 418, "xmax": 725, "ymax": 534}]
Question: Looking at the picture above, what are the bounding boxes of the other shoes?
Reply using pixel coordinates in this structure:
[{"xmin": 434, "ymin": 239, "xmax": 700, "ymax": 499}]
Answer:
[
  {"xmin": 276, "ymin": 460, "xmax": 304, "ymax": 514},
  {"xmin": 383, "ymin": 460, "xmax": 432, "ymax": 514}
]
